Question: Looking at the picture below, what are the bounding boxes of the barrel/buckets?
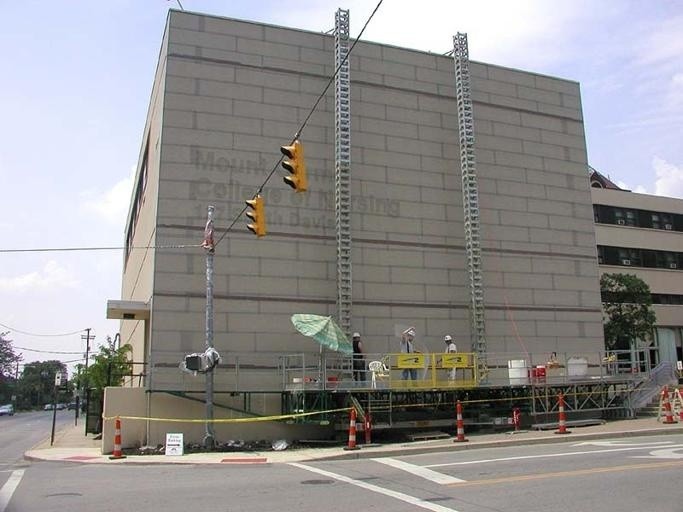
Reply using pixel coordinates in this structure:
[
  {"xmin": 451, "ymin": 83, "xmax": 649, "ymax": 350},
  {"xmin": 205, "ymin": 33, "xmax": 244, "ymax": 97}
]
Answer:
[
  {"xmin": 566, "ymin": 355, "xmax": 589, "ymax": 382},
  {"xmin": 507, "ymin": 360, "xmax": 529, "ymax": 386},
  {"xmin": 529, "ymin": 368, "xmax": 547, "ymax": 384}
]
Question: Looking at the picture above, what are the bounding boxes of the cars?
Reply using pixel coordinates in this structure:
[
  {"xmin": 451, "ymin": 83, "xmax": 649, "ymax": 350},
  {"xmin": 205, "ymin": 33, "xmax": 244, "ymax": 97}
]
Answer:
[{"xmin": 43, "ymin": 399, "xmax": 82, "ymax": 411}]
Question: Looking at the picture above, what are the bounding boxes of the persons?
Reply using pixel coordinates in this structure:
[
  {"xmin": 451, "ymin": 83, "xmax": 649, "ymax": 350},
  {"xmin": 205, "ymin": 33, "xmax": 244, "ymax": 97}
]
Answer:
[
  {"xmin": 434, "ymin": 335, "xmax": 456, "ymax": 380},
  {"xmin": 351, "ymin": 332, "xmax": 367, "ymax": 380},
  {"xmin": 400, "ymin": 327, "xmax": 418, "ymax": 380}
]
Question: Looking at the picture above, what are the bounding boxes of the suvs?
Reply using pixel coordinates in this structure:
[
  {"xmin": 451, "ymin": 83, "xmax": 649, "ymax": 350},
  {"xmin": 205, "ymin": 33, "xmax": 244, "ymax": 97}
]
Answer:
[{"xmin": 0, "ymin": 404, "xmax": 14, "ymax": 415}]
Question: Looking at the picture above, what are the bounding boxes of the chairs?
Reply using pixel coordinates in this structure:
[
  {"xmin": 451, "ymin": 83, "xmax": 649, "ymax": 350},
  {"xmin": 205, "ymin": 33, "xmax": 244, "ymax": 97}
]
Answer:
[{"xmin": 368, "ymin": 361, "xmax": 389, "ymax": 389}]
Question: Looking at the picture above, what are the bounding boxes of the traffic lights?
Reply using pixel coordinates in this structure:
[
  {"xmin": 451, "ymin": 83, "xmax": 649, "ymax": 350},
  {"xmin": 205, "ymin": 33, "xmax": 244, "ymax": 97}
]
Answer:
[
  {"xmin": 280, "ymin": 139, "xmax": 305, "ymax": 193},
  {"xmin": 245, "ymin": 194, "xmax": 266, "ymax": 238},
  {"xmin": 186, "ymin": 355, "xmax": 202, "ymax": 370}
]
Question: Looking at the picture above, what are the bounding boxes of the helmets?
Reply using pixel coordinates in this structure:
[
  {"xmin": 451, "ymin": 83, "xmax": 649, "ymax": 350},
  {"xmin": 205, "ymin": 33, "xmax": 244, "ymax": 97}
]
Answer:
[
  {"xmin": 408, "ymin": 330, "xmax": 416, "ymax": 336},
  {"xmin": 353, "ymin": 332, "xmax": 360, "ymax": 338},
  {"xmin": 445, "ymin": 335, "xmax": 452, "ymax": 341}
]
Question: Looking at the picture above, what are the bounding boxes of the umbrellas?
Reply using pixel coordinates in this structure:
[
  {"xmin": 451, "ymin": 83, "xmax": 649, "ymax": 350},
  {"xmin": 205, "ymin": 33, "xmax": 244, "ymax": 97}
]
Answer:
[{"xmin": 290, "ymin": 311, "xmax": 353, "ymax": 381}]
